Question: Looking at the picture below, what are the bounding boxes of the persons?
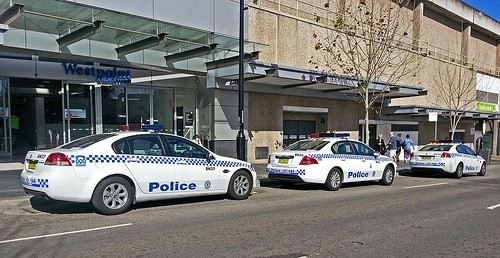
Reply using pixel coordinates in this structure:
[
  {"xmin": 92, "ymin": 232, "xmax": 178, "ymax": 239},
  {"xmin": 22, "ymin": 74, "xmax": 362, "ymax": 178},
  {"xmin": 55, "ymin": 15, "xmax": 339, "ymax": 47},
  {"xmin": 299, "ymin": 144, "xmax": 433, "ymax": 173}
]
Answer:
[
  {"xmin": 189, "ymin": 135, "xmax": 203, "ymax": 152},
  {"xmin": 6, "ymin": 111, "xmax": 21, "ymax": 147},
  {"xmin": 374, "ymin": 134, "xmax": 387, "ymax": 155},
  {"xmin": 386, "ymin": 131, "xmax": 397, "ymax": 162},
  {"xmin": 395, "ymin": 133, "xmax": 415, "ymax": 163}
]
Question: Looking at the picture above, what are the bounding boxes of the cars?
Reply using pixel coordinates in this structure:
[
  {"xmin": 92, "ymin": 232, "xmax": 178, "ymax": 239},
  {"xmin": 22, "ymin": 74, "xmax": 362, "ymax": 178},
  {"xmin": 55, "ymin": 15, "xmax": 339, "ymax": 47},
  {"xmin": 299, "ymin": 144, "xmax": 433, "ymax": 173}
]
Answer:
[
  {"xmin": 20, "ymin": 125, "xmax": 261, "ymax": 215},
  {"xmin": 409, "ymin": 138, "xmax": 487, "ymax": 179},
  {"xmin": 265, "ymin": 132, "xmax": 398, "ymax": 191}
]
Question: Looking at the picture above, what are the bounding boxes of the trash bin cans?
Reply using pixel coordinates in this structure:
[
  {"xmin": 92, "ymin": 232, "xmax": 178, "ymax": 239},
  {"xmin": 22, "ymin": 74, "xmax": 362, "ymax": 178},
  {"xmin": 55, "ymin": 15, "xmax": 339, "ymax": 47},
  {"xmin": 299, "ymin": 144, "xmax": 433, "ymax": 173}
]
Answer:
[
  {"xmin": 254, "ymin": 146, "xmax": 269, "ymax": 159},
  {"xmin": 481, "ymin": 149, "xmax": 491, "ymax": 164}
]
